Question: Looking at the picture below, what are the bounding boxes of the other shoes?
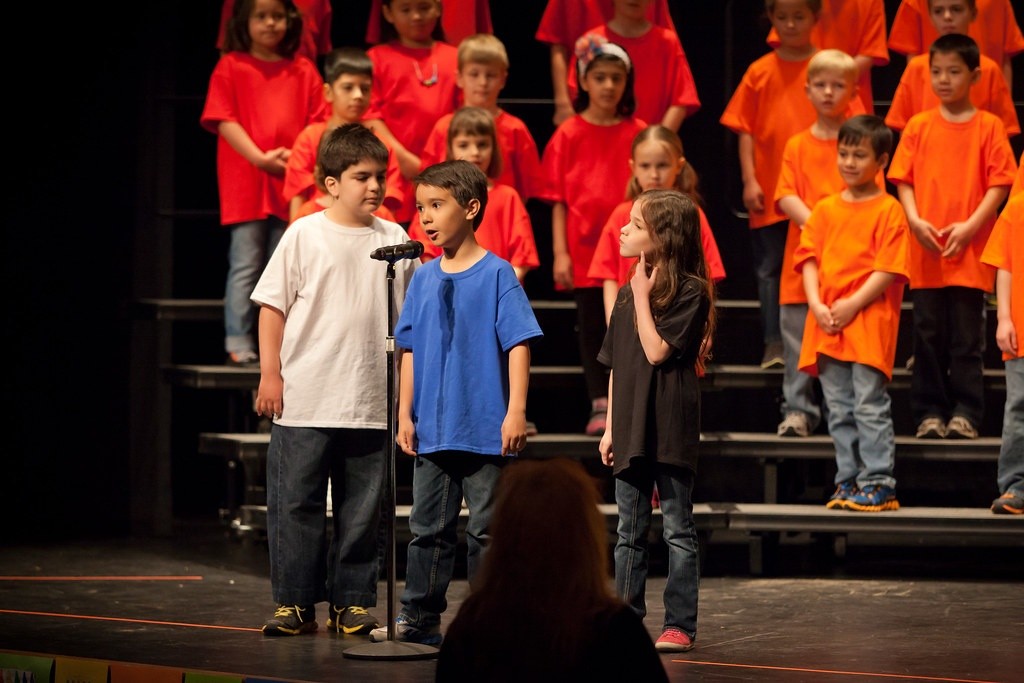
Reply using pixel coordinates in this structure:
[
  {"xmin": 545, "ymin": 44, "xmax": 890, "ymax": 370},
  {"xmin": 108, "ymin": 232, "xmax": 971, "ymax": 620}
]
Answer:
[
  {"xmin": 651, "ymin": 487, "xmax": 663, "ymax": 512},
  {"xmin": 525, "ymin": 418, "xmax": 537, "ymax": 436}
]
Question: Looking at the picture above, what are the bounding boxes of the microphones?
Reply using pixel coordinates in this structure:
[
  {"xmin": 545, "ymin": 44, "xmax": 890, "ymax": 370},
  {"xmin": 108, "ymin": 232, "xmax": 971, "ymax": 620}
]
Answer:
[{"xmin": 370, "ymin": 240, "xmax": 425, "ymax": 262}]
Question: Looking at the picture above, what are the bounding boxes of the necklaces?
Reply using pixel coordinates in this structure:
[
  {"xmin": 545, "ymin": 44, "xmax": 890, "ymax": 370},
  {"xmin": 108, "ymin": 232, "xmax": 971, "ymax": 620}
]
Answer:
[{"xmin": 412, "ymin": 56, "xmax": 437, "ymax": 85}]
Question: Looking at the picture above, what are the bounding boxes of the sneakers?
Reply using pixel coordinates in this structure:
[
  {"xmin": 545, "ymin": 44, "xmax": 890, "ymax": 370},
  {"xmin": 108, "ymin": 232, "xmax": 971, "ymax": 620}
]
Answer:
[
  {"xmin": 944, "ymin": 416, "xmax": 979, "ymax": 439},
  {"xmin": 261, "ymin": 602, "xmax": 318, "ymax": 636},
  {"xmin": 369, "ymin": 615, "xmax": 440, "ymax": 642},
  {"xmin": 654, "ymin": 627, "xmax": 696, "ymax": 652},
  {"xmin": 586, "ymin": 403, "xmax": 608, "ymax": 434},
  {"xmin": 825, "ymin": 478, "xmax": 859, "ymax": 509},
  {"xmin": 991, "ymin": 489, "xmax": 1024, "ymax": 514},
  {"xmin": 759, "ymin": 339, "xmax": 786, "ymax": 368},
  {"xmin": 327, "ymin": 601, "xmax": 379, "ymax": 634},
  {"xmin": 916, "ymin": 417, "xmax": 944, "ymax": 439},
  {"xmin": 778, "ymin": 409, "xmax": 812, "ymax": 437},
  {"xmin": 843, "ymin": 483, "xmax": 900, "ymax": 511},
  {"xmin": 228, "ymin": 350, "xmax": 260, "ymax": 369}
]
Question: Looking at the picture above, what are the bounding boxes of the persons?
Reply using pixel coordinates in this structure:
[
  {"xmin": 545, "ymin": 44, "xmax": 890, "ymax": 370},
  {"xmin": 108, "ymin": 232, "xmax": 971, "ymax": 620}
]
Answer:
[
  {"xmin": 433, "ymin": 461, "xmax": 670, "ymax": 683},
  {"xmin": 886, "ymin": 33, "xmax": 1018, "ymax": 441},
  {"xmin": 368, "ymin": 158, "xmax": 544, "ymax": 646},
  {"xmin": 766, "ymin": 0, "xmax": 889, "ymax": 115},
  {"xmin": 409, "ymin": 106, "xmax": 541, "ymax": 436},
  {"xmin": 793, "ymin": 115, "xmax": 911, "ymax": 511},
  {"xmin": 535, "ymin": 33, "xmax": 649, "ymax": 436},
  {"xmin": 535, "ymin": 0, "xmax": 701, "ymax": 133},
  {"xmin": 586, "ymin": 125, "xmax": 725, "ymax": 510},
  {"xmin": 283, "ymin": 48, "xmax": 420, "ymax": 510},
  {"xmin": 596, "ymin": 190, "xmax": 717, "ymax": 652},
  {"xmin": 361, "ymin": 0, "xmax": 493, "ymax": 235},
  {"xmin": 216, "ymin": 0, "xmax": 332, "ymax": 84},
  {"xmin": 200, "ymin": 0, "xmax": 323, "ymax": 366},
  {"xmin": 250, "ymin": 123, "xmax": 424, "ymax": 636},
  {"xmin": 425, "ymin": 34, "xmax": 539, "ymax": 206},
  {"xmin": 978, "ymin": 150, "xmax": 1024, "ymax": 516},
  {"xmin": 884, "ymin": 0, "xmax": 1021, "ymax": 371},
  {"xmin": 720, "ymin": 0, "xmax": 866, "ymax": 368},
  {"xmin": 774, "ymin": 48, "xmax": 885, "ymax": 437},
  {"xmin": 886, "ymin": 0, "xmax": 1024, "ymax": 98}
]
